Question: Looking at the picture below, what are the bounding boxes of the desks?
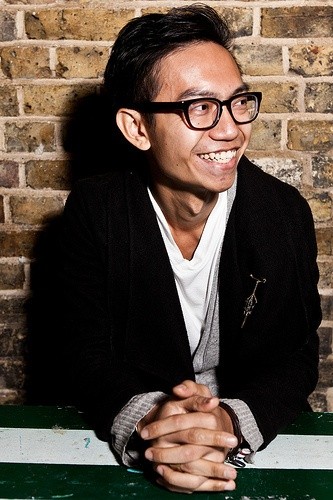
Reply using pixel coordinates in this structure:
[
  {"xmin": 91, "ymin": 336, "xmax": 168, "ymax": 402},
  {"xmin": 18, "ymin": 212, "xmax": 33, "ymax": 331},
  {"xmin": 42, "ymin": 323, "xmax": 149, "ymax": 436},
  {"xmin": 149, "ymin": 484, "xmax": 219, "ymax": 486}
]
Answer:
[{"xmin": 0, "ymin": 405, "xmax": 333, "ymax": 500}]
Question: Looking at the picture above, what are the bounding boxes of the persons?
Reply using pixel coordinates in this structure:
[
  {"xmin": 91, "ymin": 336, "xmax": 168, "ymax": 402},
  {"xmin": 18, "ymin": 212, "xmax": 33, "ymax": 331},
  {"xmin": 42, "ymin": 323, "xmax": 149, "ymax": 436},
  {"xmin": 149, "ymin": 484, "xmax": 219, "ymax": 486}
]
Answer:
[{"xmin": 21, "ymin": 2, "xmax": 323, "ymax": 494}]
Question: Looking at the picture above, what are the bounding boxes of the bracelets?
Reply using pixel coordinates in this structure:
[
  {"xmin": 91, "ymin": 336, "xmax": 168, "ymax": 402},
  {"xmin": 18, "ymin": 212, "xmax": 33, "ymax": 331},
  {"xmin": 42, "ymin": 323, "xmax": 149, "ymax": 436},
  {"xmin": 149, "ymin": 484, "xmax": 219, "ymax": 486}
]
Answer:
[{"xmin": 218, "ymin": 401, "xmax": 242, "ymax": 462}]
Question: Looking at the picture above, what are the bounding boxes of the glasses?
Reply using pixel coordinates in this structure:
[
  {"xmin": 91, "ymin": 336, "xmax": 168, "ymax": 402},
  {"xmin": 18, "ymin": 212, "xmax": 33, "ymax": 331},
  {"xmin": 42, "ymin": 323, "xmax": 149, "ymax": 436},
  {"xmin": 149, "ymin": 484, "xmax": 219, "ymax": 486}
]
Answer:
[{"xmin": 138, "ymin": 91, "xmax": 263, "ymax": 130}]
